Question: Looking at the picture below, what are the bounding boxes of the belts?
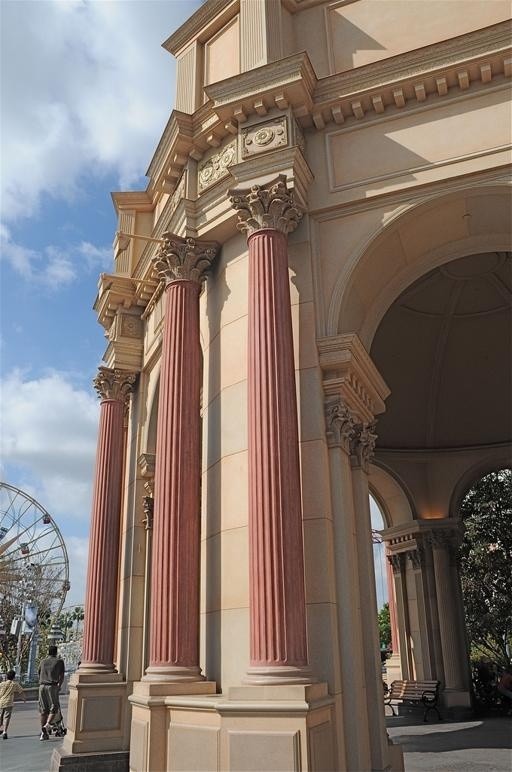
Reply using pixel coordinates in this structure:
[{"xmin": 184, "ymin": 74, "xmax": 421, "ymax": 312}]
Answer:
[{"xmin": 41, "ymin": 683, "xmax": 52, "ymax": 685}]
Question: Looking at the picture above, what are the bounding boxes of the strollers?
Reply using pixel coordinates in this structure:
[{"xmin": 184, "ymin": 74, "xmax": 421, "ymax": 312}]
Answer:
[{"xmin": 48, "ymin": 708, "xmax": 67, "ymax": 737}]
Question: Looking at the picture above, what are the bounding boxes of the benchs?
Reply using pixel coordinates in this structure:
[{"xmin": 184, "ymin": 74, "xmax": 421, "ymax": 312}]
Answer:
[{"xmin": 384, "ymin": 678, "xmax": 443, "ymax": 724}]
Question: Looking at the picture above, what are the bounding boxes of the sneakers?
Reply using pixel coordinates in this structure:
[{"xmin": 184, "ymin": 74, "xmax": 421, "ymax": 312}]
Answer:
[
  {"xmin": 42, "ymin": 726, "xmax": 49, "ymax": 740},
  {"xmin": 2, "ymin": 734, "xmax": 7, "ymax": 739},
  {"xmin": 39, "ymin": 735, "xmax": 44, "ymax": 741}
]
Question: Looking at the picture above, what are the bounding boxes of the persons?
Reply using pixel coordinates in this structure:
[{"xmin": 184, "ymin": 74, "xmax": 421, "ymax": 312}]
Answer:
[
  {"xmin": 497, "ymin": 665, "xmax": 512, "ymax": 715},
  {"xmin": 37, "ymin": 646, "xmax": 65, "ymax": 741},
  {"xmin": 479, "ymin": 663, "xmax": 501, "ymax": 697},
  {"xmin": 0, "ymin": 670, "xmax": 26, "ymax": 739}
]
